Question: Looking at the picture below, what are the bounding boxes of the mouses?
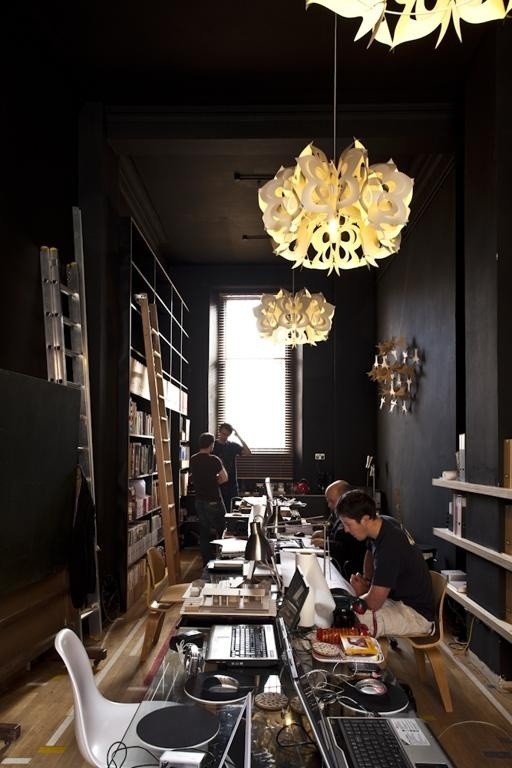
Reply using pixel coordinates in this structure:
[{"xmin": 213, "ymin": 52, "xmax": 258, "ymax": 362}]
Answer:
[
  {"xmin": 202, "ymin": 674, "xmax": 240, "ymax": 694},
  {"xmin": 294, "ymin": 531, "xmax": 304, "ymax": 536},
  {"xmin": 354, "ymin": 677, "xmax": 387, "ymax": 696}
]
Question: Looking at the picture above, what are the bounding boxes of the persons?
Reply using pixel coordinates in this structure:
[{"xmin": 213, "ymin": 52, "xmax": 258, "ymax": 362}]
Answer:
[
  {"xmin": 331, "ymin": 489, "xmax": 439, "ymax": 637},
  {"xmin": 311, "ymin": 482, "xmax": 368, "ymax": 573},
  {"xmin": 214, "ymin": 422, "xmax": 252, "ymax": 513},
  {"xmin": 190, "ymin": 431, "xmax": 231, "ymax": 569}
]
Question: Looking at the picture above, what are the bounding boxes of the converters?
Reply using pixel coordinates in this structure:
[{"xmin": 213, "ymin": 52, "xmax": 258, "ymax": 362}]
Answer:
[{"xmin": 159, "ymin": 750, "xmax": 206, "ymax": 767}]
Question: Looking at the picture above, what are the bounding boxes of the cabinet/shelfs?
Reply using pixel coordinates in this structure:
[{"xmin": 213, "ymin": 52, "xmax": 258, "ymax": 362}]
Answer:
[{"xmin": 100, "ymin": 214, "xmax": 198, "ymax": 615}]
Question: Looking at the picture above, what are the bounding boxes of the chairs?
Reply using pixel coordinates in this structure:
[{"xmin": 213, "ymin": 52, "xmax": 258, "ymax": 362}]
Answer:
[
  {"xmin": 377, "ymin": 565, "xmax": 456, "ymax": 714},
  {"xmin": 137, "ymin": 545, "xmax": 189, "ymax": 668},
  {"xmin": 50, "ymin": 626, "xmax": 190, "ymax": 766}
]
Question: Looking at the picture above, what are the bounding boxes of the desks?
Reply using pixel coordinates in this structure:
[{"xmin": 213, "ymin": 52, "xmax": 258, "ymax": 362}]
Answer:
[{"xmin": 110, "ymin": 495, "xmax": 455, "ymax": 768}]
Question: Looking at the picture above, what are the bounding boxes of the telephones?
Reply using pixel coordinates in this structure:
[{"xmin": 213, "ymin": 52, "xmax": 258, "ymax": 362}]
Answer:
[{"xmin": 326, "ymin": 595, "xmax": 367, "ymax": 629}]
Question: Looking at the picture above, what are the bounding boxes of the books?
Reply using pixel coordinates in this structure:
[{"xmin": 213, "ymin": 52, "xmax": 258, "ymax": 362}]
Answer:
[
  {"xmin": 446, "ymin": 491, "xmax": 466, "ymax": 536},
  {"xmin": 439, "ymin": 569, "xmax": 468, "ymax": 594},
  {"xmin": 125, "ymin": 400, "xmax": 188, "ymax": 608}
]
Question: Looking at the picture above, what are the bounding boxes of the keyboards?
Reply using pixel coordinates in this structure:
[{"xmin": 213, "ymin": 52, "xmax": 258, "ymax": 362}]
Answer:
[{"xmin": 301, "ymin": 537, "xmax": 320, "ymax": 548}]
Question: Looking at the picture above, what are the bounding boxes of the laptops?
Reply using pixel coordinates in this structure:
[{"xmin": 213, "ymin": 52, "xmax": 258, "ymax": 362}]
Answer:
[
  {"xmin": 205, "ymin": 564, "xmax": 310, "ymax": 667},
  {"xmin": 279, "ymin": 616, "xmax": 458, "ymax": 767}
]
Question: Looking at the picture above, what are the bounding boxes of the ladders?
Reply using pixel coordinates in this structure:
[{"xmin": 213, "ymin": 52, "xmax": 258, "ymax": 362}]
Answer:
[
  {"xmin": 41, "ymin": 247, "xmax": 103, "ymax": 643},
  {"xmin": 140, "ymin": 295, "xmax": 181, "ymax": 586}
]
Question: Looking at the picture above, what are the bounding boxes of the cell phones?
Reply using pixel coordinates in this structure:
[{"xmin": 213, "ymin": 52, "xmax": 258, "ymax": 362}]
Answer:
[{"xmin": 348, "ymin": 662, "xmax": 381, "ymax": 673}]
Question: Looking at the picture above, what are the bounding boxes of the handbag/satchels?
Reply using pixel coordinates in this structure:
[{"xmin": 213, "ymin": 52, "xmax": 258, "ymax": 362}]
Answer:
[{"xmin": 334, "ymin": 595, "xmax": 366, "ymax": 628}]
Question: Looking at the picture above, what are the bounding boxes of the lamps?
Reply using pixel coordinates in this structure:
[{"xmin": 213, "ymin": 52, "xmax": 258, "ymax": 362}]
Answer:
[
  {"xmin": 256, "ymin": 0, "xmax": 418, "ymax": 276},
  {"xmin": 302, "ymin": 0, "xmax": 512, "ymax": 53},
  {"xmin": 250, "ymin": 261, "xmax": 337, "ymax": 346}
]
